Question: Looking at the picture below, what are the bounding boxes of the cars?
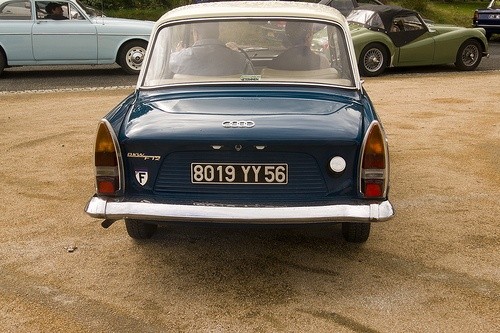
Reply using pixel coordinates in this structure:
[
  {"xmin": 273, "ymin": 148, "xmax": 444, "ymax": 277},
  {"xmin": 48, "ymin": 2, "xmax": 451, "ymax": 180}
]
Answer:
[
  {"xmin": 471, "ymin": 0, "xmax": 500, "ymax": 40},
  {"xmin": 0, "ymin": 0, "xmax": 180, "ymax": 76},
  {"xmin": 261, "ymin": 0, "xmax": 434, "ymax": 49},
  {"xmin": 82, "ymin": 1, "xmax": 397, "ymax": 244}
]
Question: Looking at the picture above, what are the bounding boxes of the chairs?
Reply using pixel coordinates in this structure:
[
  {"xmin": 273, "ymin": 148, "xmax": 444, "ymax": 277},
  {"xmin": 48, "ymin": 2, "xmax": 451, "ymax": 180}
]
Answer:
[{"xmin": 260, "ymin": 67, "xmax": 338, "ymax": 80}]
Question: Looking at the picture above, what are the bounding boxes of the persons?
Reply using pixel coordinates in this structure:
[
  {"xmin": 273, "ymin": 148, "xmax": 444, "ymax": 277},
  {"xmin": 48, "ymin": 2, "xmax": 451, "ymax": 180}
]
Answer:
[
  {"xmin": 58, "ymin": 3, "xmax": 69, "ymax": 19},
  {"xmin": 44, "ymin": 3, "xmax": 66, "ymax": 20},
  {"xmin": 169, "ymin": 22, "xmax": 256, "ymax": 76},
  {"xmin": 267, "ymin": 21, "xmax": 329, "ymax": 70}
]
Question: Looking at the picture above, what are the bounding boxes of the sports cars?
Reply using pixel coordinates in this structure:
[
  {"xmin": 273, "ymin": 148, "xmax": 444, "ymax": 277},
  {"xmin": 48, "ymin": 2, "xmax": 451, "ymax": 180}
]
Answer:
[{"xmin": 311, "ymin": 4, "xmax": 491, "ymax": 77}]
